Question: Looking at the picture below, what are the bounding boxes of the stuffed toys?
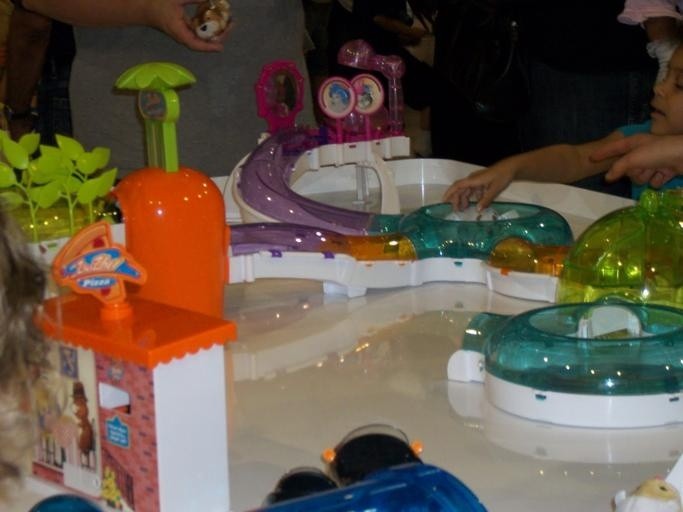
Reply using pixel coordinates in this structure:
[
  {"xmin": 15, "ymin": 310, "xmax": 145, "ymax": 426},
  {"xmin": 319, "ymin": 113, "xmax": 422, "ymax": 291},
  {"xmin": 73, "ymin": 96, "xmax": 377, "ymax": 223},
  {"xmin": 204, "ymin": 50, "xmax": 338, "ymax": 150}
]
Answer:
[{"xmin": 189, "ymin": 0, "xmax": 230, "ymax": 41}]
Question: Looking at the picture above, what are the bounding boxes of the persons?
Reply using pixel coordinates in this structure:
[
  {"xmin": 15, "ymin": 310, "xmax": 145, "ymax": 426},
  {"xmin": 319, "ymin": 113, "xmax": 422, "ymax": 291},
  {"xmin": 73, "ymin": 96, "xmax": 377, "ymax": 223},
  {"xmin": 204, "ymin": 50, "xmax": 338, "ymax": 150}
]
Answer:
[
  {"xmin": 590, "ymin": 133, "xmax": 683, "ymax": 190},
  {"xmin": 617, "ymin": 0, "xmax": 683, "ymax": 82},
  {"xmin": 21, "ymin": 0, "xmax": 319, "ymax": 184},
  {"xmin": 441, "ymin": 46, "xmax": 683, "ymax": 214}
]
